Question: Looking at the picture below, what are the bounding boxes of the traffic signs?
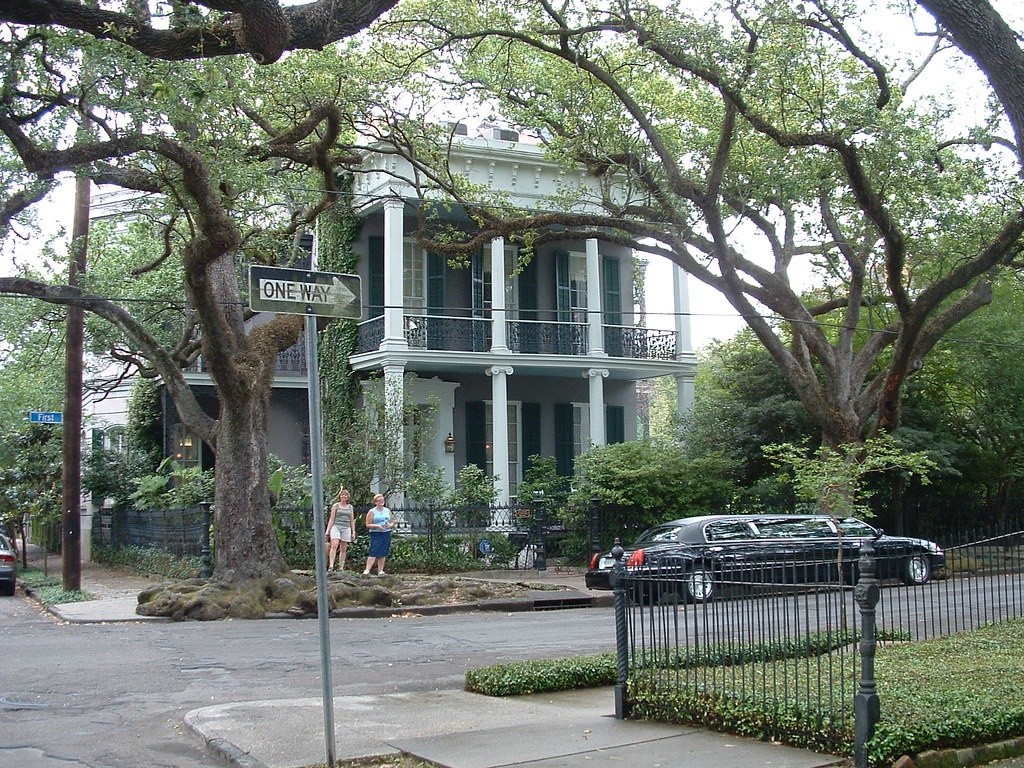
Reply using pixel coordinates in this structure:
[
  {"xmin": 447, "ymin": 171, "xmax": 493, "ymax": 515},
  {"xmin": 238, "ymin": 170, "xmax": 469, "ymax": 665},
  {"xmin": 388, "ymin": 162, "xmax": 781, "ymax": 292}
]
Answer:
[{"xmin": 248, "ymin": 265, "xmax": 363, "ymax": 320}]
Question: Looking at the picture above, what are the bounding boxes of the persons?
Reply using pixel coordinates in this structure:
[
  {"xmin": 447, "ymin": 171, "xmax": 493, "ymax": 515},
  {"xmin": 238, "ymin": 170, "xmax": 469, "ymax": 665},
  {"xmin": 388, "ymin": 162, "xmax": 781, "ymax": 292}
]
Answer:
[
  {"xmin": 325, "ymin": 490, "xmax": 355, "ymax": 571},
  {"xmin": 363, "ymin": 494, "xmax": 394, "ymax": 576}
]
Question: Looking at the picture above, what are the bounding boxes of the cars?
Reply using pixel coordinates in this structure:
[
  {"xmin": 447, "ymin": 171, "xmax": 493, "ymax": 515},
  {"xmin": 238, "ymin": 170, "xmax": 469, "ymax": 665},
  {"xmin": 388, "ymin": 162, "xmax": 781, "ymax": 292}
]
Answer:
[
  {"xmin": 0, "ymin": 532, "xmax": 19, "ymax": 595},
  {"xmin": 584, "ymin": 511, "xmax": 947, "ymax": 605}
]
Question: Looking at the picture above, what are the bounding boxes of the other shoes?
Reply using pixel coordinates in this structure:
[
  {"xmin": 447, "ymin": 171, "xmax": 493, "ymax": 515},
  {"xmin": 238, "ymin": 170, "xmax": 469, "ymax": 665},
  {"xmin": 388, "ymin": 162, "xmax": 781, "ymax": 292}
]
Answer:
[
  {"xmin": 363, "ymin": 569, "xmax": 369, "ymax": 575},
  {"xmin": 378, "ymin": 570, "xmax": 385, "ymax": 576},
  {"xmin": 328, "ymin": 567, "xmax": 333, "ymax": 572}
]
